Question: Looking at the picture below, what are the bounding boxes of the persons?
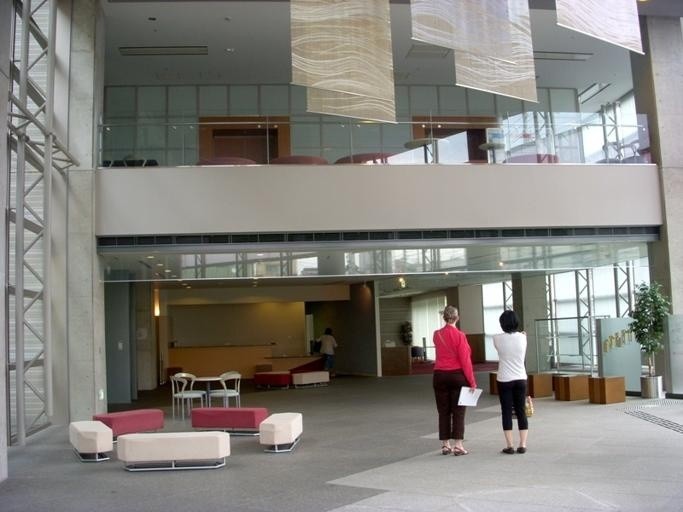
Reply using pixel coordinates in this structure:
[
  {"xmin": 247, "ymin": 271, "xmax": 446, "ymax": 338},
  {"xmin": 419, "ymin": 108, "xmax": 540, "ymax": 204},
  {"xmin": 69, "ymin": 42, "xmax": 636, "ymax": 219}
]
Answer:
[
  {"xmin": 493, "ymin": 310, "xmax": 529, "ymax": 454},
  {"xmin": 433, "ymin": 305, "xmax": 477, "ymax": 454},
  {"xmin": 316, "ymin": 328, "xmax": 339, "ymax": 371}
]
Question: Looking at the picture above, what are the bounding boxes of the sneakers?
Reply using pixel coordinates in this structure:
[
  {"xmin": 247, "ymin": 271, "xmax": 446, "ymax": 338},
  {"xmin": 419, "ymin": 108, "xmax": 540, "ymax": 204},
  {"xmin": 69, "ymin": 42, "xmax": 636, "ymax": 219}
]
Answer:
[
  {"xmin": 517, "ymin": 447, "xmax": 527, "ymax": 454},
  {"xmin": 502, "ymin": 447, "xmax": 514, "ymax": 454}
]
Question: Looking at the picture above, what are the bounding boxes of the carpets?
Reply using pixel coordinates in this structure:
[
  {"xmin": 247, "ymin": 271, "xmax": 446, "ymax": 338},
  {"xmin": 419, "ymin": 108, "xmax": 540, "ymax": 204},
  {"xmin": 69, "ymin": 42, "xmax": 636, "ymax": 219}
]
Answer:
[{"xmin": 411, "ymin": 356, "xmax": 499, "ymax": 374}]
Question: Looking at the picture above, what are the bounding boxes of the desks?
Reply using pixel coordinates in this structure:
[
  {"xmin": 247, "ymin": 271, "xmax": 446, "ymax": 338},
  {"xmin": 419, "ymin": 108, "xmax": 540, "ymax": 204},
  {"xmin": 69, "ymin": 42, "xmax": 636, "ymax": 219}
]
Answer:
[{"xmin": 196, "ymin": 135, "xmax": 650, "ymax": 165}]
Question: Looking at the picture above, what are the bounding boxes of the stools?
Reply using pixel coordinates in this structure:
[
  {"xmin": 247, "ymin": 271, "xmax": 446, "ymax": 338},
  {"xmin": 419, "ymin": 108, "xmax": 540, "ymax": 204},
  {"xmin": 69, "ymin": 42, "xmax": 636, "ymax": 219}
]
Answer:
[{"xmin": 411, "ymin": 346, "xmax": 424, "ymax": 364}]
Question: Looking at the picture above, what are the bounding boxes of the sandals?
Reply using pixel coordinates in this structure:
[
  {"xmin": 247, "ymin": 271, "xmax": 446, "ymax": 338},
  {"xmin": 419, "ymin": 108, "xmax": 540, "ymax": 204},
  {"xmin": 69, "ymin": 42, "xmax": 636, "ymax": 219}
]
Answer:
[
  {"xmin": 454, "ymin": 446, "xmax": 469, "ymax": 456},
  {"xmin": 442, "ymin": 446, "xmax": 454, "ymax": 455}
]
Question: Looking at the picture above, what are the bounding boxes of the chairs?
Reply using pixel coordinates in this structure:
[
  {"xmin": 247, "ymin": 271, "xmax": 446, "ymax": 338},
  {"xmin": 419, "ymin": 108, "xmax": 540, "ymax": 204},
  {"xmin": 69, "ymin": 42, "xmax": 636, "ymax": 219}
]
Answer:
[{"xmin": 169, "ymin": 369, "xmax": 241, "ymax": 419}]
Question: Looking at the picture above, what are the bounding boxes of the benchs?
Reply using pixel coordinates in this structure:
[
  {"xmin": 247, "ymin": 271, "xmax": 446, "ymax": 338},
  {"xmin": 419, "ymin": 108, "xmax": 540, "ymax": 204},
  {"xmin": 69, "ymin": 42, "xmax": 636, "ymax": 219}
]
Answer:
[
  {"xmin": 257, "ymin": 409, "xmax": 304, "ymax": 452},
  {"xmin": 188, "ymin": 405, "xmax": 269, "ymax": 437},
  {"xmin": 252, "ymin": 370, "xmax": 291, "ymax": 388},
  {"xmin": 114, "ymin": 430, "xmax": 233, "ymax": 473},
  {"xmin": 90, "ymin": 406, "xmax": 166, "ymax": 437},
  {"xmin": 291, "ymin": 371, "xmax": 329, "ymax": 388},
  {"xmin": 69, "ymin": 421, "xmax": 115, "ymax": 463}
]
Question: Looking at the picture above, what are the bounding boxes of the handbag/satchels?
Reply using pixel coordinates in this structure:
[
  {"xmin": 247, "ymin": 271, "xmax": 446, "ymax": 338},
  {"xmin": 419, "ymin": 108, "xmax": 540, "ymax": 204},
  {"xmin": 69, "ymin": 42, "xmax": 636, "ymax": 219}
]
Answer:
[{"xmin": 510, "ymin": 396, "xmax": 535, "ymax": 418}]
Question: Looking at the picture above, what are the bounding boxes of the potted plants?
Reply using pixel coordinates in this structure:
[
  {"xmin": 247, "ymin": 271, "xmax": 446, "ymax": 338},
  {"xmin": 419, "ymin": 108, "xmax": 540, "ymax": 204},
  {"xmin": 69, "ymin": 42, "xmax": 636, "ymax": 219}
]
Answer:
[
  {"xmin": 400, "ymin": 321, "xmax": 413, "ymax": 345},
  {"xmin": 624, "ymin": 280, "xmax": 669, "ymax": 400}
]
